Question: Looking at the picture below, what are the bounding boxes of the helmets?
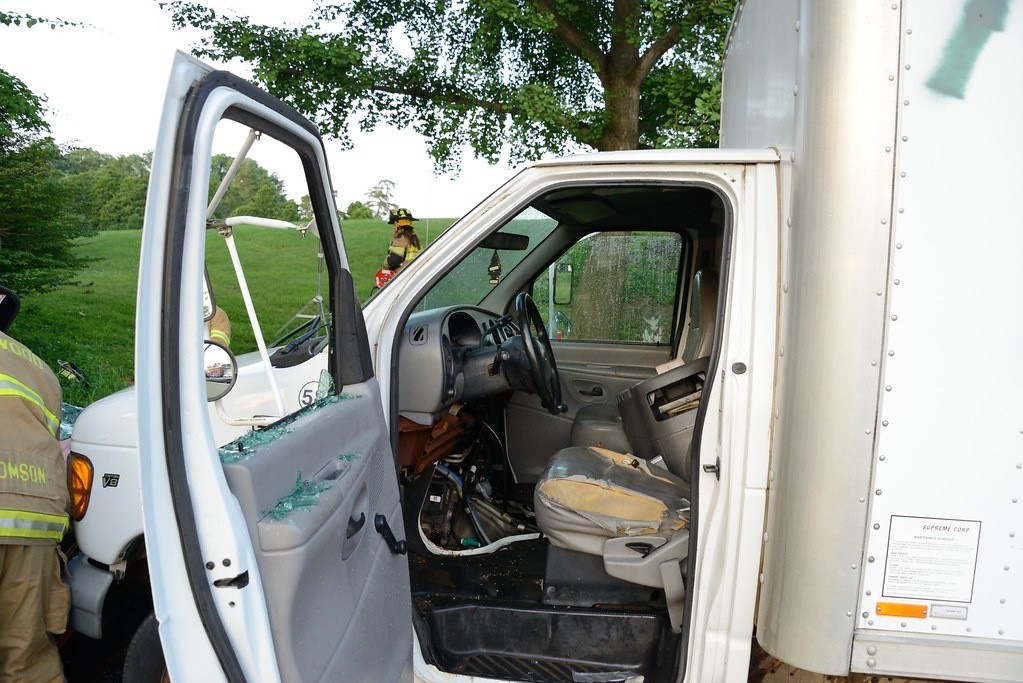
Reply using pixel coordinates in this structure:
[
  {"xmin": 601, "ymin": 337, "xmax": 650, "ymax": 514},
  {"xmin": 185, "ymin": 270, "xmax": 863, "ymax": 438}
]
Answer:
[
  {"xmin": 1, "ymin": 285, "xmax": 21, "ymax": 333},
  {"xmin": 388, "ymin": 207, "xmax": 420, "ymax": 224}
]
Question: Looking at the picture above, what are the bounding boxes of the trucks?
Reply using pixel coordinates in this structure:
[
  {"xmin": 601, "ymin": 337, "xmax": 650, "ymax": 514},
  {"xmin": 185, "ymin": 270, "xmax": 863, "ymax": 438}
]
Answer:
[{"xmin": 56, "ymin": 0, "xmax": 1023, "ymax": 683}]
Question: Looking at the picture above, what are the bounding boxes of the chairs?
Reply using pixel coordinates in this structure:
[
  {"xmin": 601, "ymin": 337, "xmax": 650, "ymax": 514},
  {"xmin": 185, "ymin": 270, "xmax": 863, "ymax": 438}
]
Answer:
[
  {"xmin": 570, "ymin": 268, "xmax": 720, "ymax": 458},
  {"xmin": 530, "ymin": 443, "xmax": 692, "ymax": 591}
]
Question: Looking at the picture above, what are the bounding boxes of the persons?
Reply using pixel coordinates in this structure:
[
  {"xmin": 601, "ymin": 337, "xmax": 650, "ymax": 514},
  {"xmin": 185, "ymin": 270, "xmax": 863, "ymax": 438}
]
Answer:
[
  {"xmin": 203, "ymin": 304, "xmax": 232, "ymax": 353},
  {"xmin": 0, "ymin": 284, "xmax": 79, "ymax": 682},
  {"xmin": 382, "ymin": 208, "xmax": 420, "ymax": 272}
]
